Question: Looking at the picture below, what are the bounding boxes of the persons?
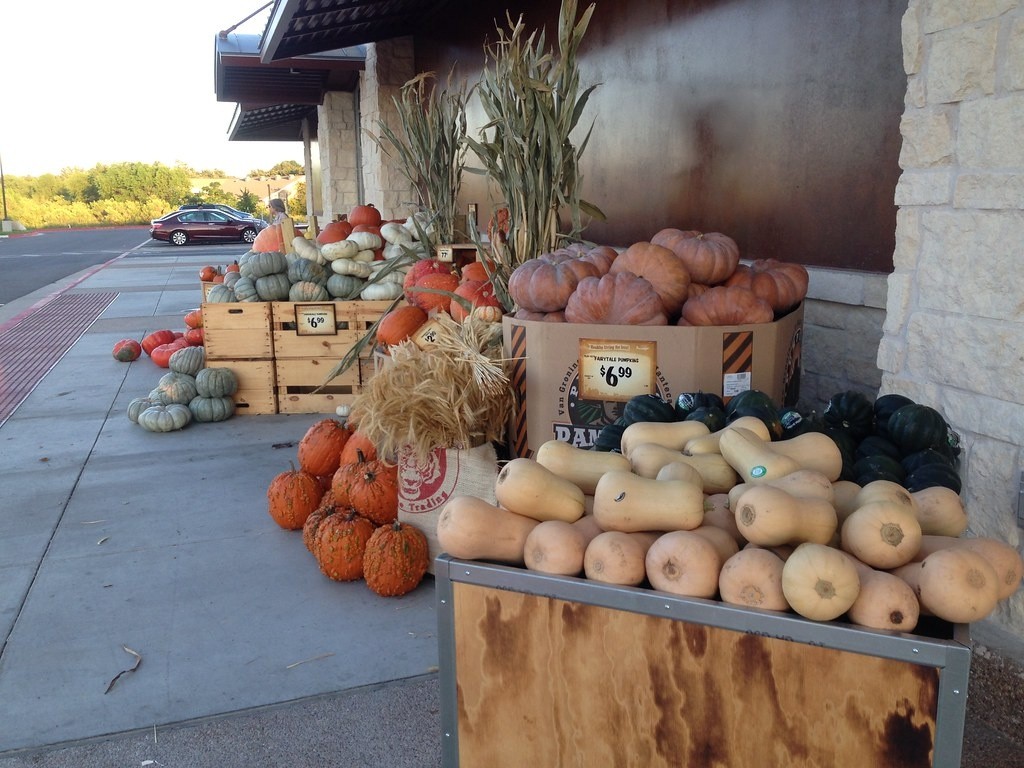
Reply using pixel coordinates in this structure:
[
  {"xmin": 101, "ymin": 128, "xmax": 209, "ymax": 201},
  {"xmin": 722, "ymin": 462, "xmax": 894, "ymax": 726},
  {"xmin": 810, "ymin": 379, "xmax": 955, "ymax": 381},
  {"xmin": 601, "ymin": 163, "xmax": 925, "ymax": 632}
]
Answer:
[
  {"xmin": 193, "ymin": 204, "xmax": 205, "ymax": 222},
  {"xmin": 269, "ymin": 198, "xmax": 289, "ymax": 226}
]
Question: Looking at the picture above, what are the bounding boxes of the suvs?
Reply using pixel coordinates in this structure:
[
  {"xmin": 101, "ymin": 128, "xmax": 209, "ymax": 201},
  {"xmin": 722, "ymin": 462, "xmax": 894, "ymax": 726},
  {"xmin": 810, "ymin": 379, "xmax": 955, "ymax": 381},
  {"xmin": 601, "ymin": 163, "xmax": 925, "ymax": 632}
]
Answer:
[{"xmin": 180, "ymin": 205, "xmax": 255, "ymax": 220}]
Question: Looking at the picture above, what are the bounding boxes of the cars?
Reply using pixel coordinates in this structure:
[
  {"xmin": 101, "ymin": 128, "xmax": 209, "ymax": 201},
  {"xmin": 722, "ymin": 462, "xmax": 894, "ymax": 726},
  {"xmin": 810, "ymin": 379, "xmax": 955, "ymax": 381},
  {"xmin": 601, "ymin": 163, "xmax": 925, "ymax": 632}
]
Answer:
[
  {"xmin": 161, "ymin": 210, "xmax": 260, "ymax": 222},
  {"xmin": 148, "ymin": 209, "xmax": 269, "ymax": 246}
]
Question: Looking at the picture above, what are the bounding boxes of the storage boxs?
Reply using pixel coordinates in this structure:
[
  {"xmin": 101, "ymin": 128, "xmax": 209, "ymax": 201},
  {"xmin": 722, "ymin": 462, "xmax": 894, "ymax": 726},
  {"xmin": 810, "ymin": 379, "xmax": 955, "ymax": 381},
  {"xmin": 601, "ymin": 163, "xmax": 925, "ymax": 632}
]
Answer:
[
  {"xmin": 434, "ymin": 244, "xmax": 491, "ymax": 269},
  {"xmin": 355, "ymin": 300, "xmax": 407, "ymax": 353},
  {"xmin": 497, "ymin": 292, "xmax": 805, "ymax": 461},
  {"xmin": 271, "ymin": 300, "xmax": 358, "ymax": 358},
  {"xmin": 204, "ymin": 356, "xmax": 277, "ymax": 415},
  {"xmin": 358, "ymin": 355, "xmax": 375, "ymax": 388},
  {"xmin": 197, "ymin": 299, "xmax": 274, "ymax": 361},
  {"xmin": 274, "ymin": 356, "xmax": 363, "ymax": 414}
]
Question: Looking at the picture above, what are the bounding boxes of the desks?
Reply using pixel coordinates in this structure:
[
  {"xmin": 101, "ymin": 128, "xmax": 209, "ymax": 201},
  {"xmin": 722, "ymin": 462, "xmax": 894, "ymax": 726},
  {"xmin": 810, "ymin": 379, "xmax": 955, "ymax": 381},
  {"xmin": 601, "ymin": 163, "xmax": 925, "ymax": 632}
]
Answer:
[{"xmin": 430, "ymin": 552, "xmax": 975, "ymax": 768}]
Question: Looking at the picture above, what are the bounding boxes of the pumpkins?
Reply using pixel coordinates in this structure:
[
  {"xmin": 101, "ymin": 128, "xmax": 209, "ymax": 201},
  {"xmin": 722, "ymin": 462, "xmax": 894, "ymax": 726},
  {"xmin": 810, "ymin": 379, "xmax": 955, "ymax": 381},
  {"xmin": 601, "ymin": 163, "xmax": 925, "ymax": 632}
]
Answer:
[{"xmin": 112, "ymin": 201, "xmax": 1023, "ymax": 633}]
